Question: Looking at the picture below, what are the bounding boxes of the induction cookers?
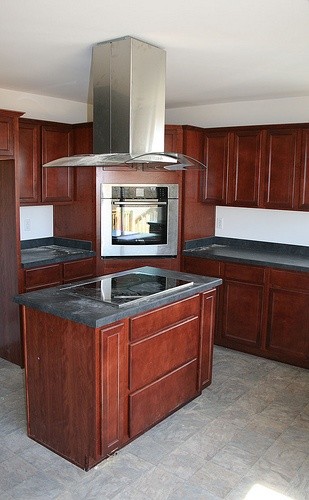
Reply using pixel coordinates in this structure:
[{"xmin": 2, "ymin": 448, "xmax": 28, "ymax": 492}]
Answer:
[{"xmin": 58, "ymin": 272, "xmax": 194, "ymax": 309}]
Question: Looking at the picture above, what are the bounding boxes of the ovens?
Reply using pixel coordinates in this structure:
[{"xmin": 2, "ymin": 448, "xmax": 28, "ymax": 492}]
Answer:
[{"xmin": 99, "ymin": 183, "xmax": 179, "ymax": 260}]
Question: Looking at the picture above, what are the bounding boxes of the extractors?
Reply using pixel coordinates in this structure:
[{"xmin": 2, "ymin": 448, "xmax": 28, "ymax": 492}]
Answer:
[{"xmin": 40, "ymin": 36, "xmax": 210, "ymax": 175}]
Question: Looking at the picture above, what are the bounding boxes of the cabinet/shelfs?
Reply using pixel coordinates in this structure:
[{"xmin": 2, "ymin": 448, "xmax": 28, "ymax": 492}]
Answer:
[
  {"xmin": 263, "ymin": 268, "xmax": 309, "ymax": 369},
  {"xmin": 18, "ymin": 118, "xmax": 40, "ymax": 208},
  {"xmin": 11, "ymin": 265, "xmax": 224, "ymax": 472},
  {"xmin": 228, "ymin": 125, "xmax": 263, "ymax": 210},
  {"xmin": 40, "ymin": 120, "xmax": 76, "ymax": 207},
  {"xmin": 1, "ymin": 264, "xmax": 64, "ymax": 369},
  {"xmin": 261, "ymin": 123, "xmax": 299, "ymax": 211},
  {"xmin": 199, "ymin": 126, "xmax": 229, "ymax": 207},
  {"xmin": 221, "ymin": 263, "xmax": 265, "ymax": 357},
  {"xmin": 181, "ymin": 256, "xmax": 223, "ymax": 348},
  {"xmin": 62, "ymin": 258, "xmax": 95, "ymax": 285},
  {"xmin": 297, "ymin": 123, "xmax": 308, "ymax": 212}
]
